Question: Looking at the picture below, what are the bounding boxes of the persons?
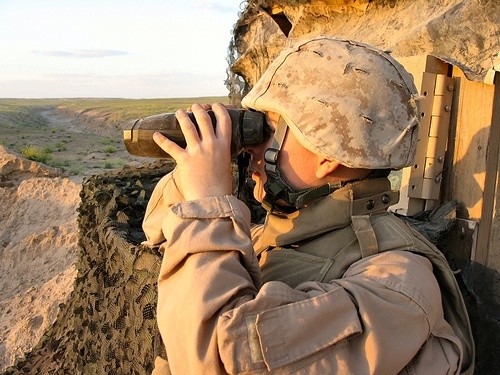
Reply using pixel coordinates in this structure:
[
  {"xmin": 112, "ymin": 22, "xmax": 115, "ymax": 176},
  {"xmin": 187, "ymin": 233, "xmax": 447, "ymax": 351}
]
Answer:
[{"xmin": 142, "ymin": 37, "xmax": 477, "ymax": 375}]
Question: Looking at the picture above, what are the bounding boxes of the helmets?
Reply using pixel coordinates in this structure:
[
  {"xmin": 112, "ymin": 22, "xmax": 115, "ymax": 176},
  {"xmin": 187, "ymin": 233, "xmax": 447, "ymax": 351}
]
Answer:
[{"xmin": 241, "ymin": 36, "xmax": 420, "ymax": 170}]
